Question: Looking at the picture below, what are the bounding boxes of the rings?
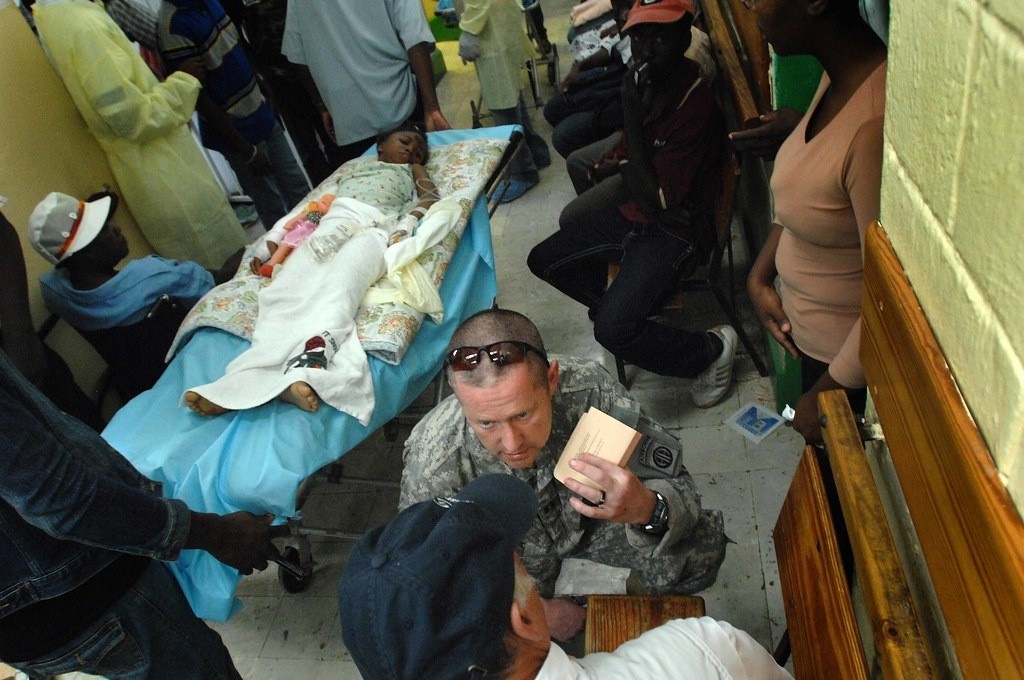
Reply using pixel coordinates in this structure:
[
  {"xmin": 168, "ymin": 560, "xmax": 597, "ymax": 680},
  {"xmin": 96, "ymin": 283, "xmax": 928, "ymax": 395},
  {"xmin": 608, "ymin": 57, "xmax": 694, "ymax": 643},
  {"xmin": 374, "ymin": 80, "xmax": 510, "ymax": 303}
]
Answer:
[{"xmin": 594, "ymin": 489, "xmax": 606, "ymax": 507}]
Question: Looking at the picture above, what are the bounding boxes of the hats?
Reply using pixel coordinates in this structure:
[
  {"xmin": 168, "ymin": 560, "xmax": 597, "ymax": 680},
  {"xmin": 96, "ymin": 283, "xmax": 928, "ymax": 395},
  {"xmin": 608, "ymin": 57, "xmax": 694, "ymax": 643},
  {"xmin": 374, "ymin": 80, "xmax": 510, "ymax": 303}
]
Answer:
[
  {"xmin": 25, "ymin": 192, "xmax": 112, "ymax": 265},
  {"xmin": 338, "ymin": 472, "xmax": 541, "ymax": 680},
  {"xmin": 621, "ymin": 0, "xmax": 697, "ymax": 33}
]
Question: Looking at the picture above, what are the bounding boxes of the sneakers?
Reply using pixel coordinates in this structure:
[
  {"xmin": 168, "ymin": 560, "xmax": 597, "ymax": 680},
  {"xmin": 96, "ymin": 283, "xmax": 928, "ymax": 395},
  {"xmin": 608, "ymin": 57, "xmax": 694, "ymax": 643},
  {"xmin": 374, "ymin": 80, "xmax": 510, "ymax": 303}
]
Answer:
[{"xmin": 692, "ymin": 323, "xmax": 739, "ymax": 409}]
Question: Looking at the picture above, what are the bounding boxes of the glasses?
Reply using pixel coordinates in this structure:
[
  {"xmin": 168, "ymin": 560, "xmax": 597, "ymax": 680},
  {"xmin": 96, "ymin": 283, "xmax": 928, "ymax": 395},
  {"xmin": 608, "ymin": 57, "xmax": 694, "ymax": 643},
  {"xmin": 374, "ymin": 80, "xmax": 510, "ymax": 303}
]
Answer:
[
  {"xmin": 443, "ymin": 339, "xmax": 552, "ymax": 372},
  {"xmin": 517, "ymin": 541, "xmax": 526, "ymax": 566},
  {"xmin": 742, "ymin": 0, "xmax": 757, "ymax": 8}
]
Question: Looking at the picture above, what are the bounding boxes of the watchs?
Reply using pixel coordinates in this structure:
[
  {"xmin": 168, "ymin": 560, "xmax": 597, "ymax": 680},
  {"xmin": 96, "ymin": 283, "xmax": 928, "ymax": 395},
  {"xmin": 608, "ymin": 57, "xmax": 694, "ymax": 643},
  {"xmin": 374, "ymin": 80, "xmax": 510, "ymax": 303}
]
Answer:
[{"xmin": 633, "ymin": 489, "xmax": 670, "ymax": 534}]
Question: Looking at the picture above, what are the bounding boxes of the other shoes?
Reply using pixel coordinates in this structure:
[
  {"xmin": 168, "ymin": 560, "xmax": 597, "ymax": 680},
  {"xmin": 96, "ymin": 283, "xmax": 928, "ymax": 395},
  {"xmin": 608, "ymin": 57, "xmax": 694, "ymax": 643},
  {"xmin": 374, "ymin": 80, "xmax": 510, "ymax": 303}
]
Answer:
[{"xmin": 491, "ymin": 174, "xmax": 539, "ymax": 202}]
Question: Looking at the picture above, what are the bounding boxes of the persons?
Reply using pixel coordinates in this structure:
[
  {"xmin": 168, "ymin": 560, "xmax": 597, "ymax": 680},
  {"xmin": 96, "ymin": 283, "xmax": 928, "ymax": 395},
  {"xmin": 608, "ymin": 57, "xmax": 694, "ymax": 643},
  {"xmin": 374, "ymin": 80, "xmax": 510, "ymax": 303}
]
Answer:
[
  {"xmin": 747, "ymin": 0, "xmax": 888, "ymax": 596},
  {"xmin": 0, "ymin": 193, "xmax": 293, "ymax": 680},
  {"xmin": 17, "ymin": 0, "xmax": 340, "ymax": 279},
  {"xmin": 278, "ymin": 0, "xmax": 459, "ymax": 153},
  {"xmin": 451, "ymin": 0, "xmax": 553, "ymax": 205},
  {"xmin": 341, "ymin": 309, "xmax": 796, "ymax": 680},
  {"xmin": 184, "ymin": 125, "xmax": 440, "ymax": 418},
  {"xmin": 521, "ymin": 0, "xmax": 806, "ymax": 408}
]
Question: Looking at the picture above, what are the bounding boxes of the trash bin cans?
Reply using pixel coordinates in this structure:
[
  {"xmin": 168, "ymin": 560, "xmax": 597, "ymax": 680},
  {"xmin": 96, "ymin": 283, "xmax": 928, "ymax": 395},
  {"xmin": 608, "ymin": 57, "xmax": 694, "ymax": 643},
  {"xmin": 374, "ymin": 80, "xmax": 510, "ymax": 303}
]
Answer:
[{"xmin": 765, "ymin": 275, "xmax": 805, "ymax": 417}]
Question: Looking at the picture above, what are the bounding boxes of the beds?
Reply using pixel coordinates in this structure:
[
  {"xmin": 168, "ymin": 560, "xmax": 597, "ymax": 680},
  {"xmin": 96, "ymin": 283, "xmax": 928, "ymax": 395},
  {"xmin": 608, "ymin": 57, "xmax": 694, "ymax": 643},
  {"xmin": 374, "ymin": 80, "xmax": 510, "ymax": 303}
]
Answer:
[{"xmin": 98, "ymin": 123, "xmax": 527, "ymax": 625}]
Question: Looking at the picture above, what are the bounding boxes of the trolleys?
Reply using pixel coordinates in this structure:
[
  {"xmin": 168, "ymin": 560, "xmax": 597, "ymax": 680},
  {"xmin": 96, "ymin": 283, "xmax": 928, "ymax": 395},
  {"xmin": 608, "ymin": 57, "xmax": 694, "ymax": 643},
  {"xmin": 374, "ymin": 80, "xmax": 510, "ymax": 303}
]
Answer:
[{"xmin": 94, "ymin": 119, "xmax": 533, "ymax": 595}]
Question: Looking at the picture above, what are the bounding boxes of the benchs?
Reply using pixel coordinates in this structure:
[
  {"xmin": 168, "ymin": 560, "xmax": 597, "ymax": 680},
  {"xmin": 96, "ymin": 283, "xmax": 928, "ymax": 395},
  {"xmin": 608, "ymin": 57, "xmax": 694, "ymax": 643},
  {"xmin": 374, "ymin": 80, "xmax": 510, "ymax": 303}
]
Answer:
[{"xmin": 584, "ymin": 389, "xmax": 937, "ymax": 680}]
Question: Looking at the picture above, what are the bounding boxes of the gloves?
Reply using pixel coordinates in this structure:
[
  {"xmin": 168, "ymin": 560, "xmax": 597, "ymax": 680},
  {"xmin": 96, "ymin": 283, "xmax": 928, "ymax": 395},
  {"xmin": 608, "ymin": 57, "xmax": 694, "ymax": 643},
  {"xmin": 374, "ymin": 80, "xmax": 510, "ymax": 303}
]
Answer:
[{"xmin": 457, "ymin": 32, "xmax": 482, "ymax": 65}]
